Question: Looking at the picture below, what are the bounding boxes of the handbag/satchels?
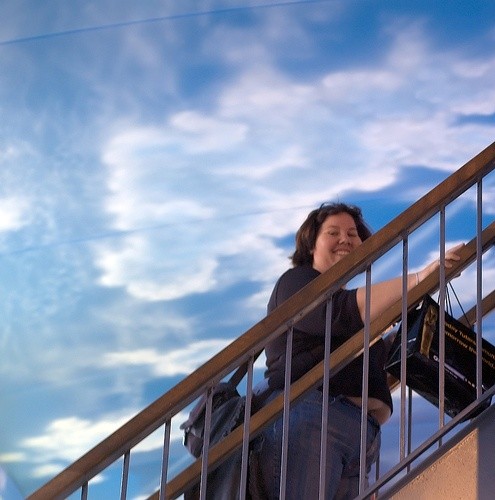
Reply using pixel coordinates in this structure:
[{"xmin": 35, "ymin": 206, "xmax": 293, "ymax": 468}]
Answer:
[
  {"xmin": 383, "ymin": 280, "xmax": 495, "ymax": 423},
  {"xmin": 180, "ymin": 381, "xmax": 251, "ymax": 500}
]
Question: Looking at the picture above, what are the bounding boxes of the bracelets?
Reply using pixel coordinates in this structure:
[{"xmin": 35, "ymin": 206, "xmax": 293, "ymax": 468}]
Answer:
[{"xmin": 415, "ymin": 272, "xmax": 420, "ymax": 286}]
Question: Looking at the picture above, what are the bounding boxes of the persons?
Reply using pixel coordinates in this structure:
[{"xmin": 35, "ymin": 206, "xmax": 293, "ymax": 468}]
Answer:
[{"xmin": 254, "ymin": 201, "xmax": 466, "ymax": 499}]
